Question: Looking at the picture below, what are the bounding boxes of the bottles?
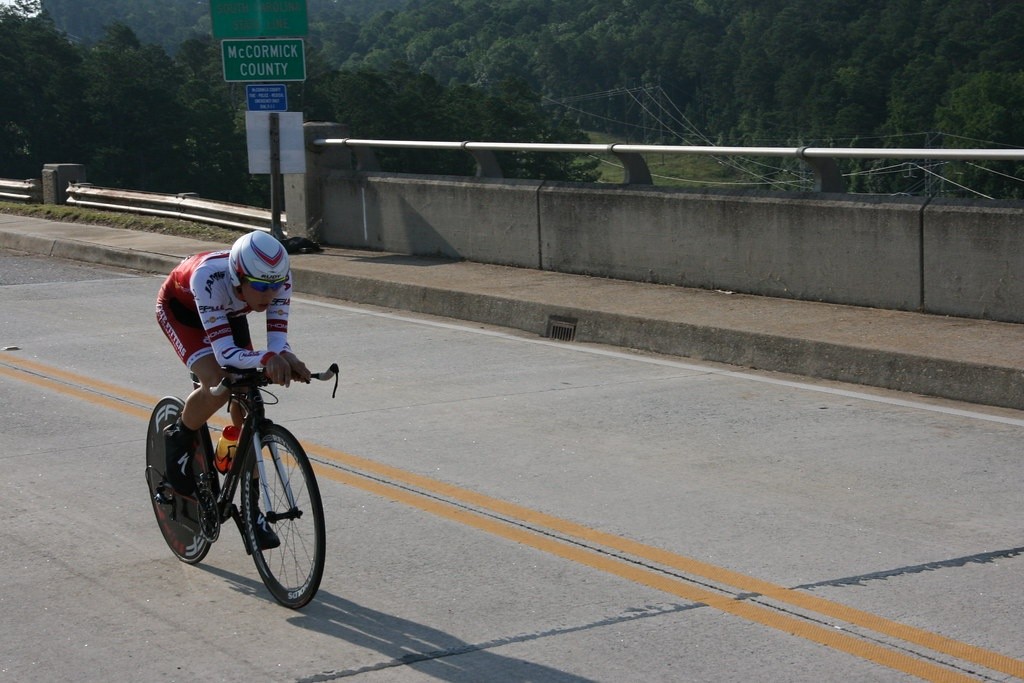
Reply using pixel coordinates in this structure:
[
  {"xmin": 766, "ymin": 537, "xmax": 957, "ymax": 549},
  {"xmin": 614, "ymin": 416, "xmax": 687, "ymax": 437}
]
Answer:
[{"xmin": 214, "ymin": 425, "xmax": 240, "ymax": 476}]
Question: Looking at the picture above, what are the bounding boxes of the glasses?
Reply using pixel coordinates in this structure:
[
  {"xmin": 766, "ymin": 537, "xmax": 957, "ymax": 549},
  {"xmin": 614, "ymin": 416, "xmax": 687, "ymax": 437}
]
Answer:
[{"xmin": 244, "ymin": 276, "xmax": 288, "ymax": 292}]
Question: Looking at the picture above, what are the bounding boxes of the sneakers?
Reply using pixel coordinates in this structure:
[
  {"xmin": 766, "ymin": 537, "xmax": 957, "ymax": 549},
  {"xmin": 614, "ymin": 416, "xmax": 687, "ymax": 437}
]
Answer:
[
  {"xmin": 240, "ymin": 510, "xmax": 281, "ymax": 549},
  {"xmin": 163, "ymin": 423, "xmax": 196, "ymax": 496}
]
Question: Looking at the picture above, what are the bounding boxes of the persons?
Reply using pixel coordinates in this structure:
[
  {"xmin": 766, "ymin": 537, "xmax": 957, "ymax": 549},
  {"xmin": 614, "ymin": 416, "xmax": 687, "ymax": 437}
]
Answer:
[{"xmin": 156, "ymin": 231, "xmax": 311, "ymax": 546}]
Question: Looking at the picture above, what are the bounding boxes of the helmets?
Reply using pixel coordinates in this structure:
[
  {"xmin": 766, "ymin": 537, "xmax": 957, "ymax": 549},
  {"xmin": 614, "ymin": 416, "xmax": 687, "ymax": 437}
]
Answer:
[{"xmin": 228, "ymin": 231, "xmax": 291, "ymax": 299}]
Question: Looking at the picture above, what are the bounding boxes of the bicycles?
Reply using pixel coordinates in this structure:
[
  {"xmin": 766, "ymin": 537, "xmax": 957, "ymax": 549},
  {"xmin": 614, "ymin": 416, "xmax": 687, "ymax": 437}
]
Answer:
[{"xmin": 145, "ymin": 363, "xmax": 339, "ymax": 609}]
